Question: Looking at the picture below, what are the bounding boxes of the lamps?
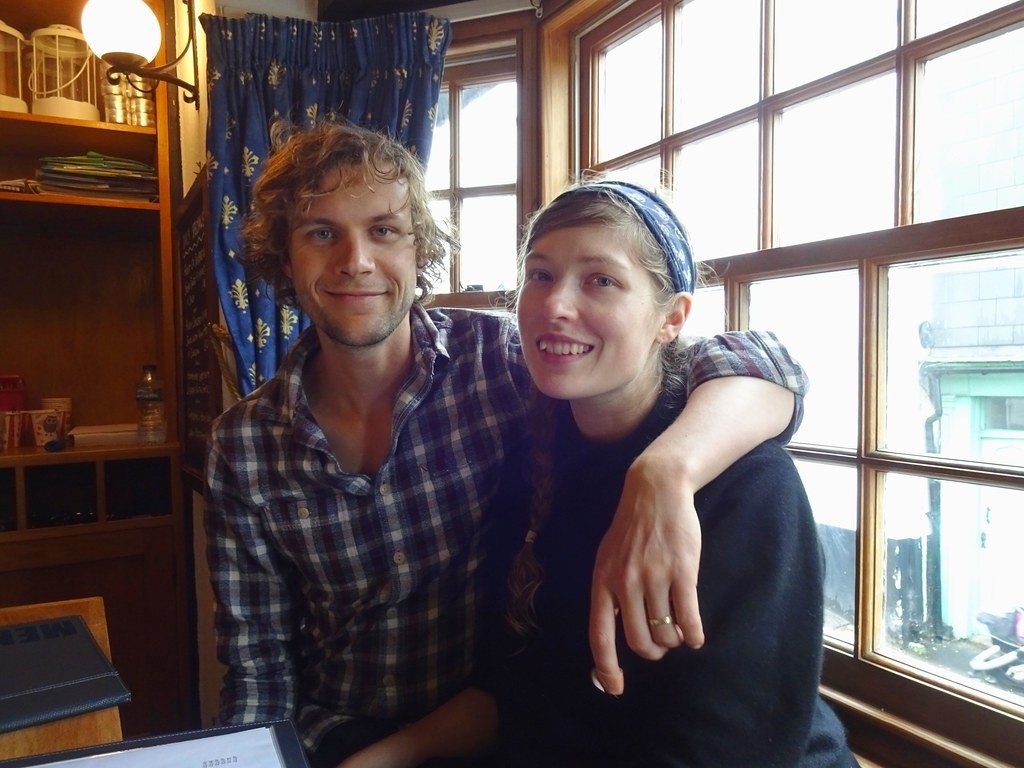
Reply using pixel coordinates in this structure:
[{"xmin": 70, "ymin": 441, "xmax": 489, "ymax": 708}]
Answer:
[{"xmin": 82, "ymin": 1, "xmax": 205, "ymax": 110}]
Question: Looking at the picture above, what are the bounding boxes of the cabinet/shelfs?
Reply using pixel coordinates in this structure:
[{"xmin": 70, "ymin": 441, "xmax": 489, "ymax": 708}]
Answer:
[{"xmin": 0, "ymin": 0, "xmax": 193, "ymax": 741}]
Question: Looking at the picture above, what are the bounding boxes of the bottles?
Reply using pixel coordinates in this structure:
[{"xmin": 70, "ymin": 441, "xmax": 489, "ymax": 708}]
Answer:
[
  {"xmin": 0, "ymin": 374, "xmax": 26, "ymax": 411},
  {"xmin": 136, "ymin": 364, "xmax": 166, "ymax": 445}
]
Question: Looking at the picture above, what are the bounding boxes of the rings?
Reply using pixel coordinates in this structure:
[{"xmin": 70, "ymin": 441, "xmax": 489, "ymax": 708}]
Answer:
[{"xmin": 649, "ymin": 616, "xmax": 674, "ymax": 625}]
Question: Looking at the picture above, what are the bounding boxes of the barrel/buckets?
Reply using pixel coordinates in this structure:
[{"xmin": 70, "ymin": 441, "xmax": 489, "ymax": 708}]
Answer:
[{"xmin": 0, "ymin": 19, "xmax": 100, "ymax": 122}]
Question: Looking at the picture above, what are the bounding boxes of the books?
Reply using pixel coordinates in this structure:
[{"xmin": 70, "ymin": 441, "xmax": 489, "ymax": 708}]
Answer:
[{"xmin": 0, "ymin": 150, "xmax": 159, "ymax": 203}]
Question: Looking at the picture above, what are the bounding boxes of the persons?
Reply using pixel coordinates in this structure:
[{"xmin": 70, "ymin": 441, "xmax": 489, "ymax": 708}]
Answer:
[
  {"xmin": 202, "ymin": 123, "xmax": 810, "ymax": 767},
  {"xmin": 340, "ymin": 182, "xmax": 864, "ymax": 768}
]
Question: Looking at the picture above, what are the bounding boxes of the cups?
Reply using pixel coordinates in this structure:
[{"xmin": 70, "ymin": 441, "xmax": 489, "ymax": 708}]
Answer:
[
  {"xmin": 0, "ymin": 411, "xmax": 23, "ymax": 449},
  {"xmin": 21, "ymin": 411, "xmax": 38, "ymax": 447},
  {"xmin": 32, "ymin": 410, "xmax": 58, "ymax": 446},
  {"xmin": 40, "ymin": 397, "xmax": 73, "ymax": 441}
]
